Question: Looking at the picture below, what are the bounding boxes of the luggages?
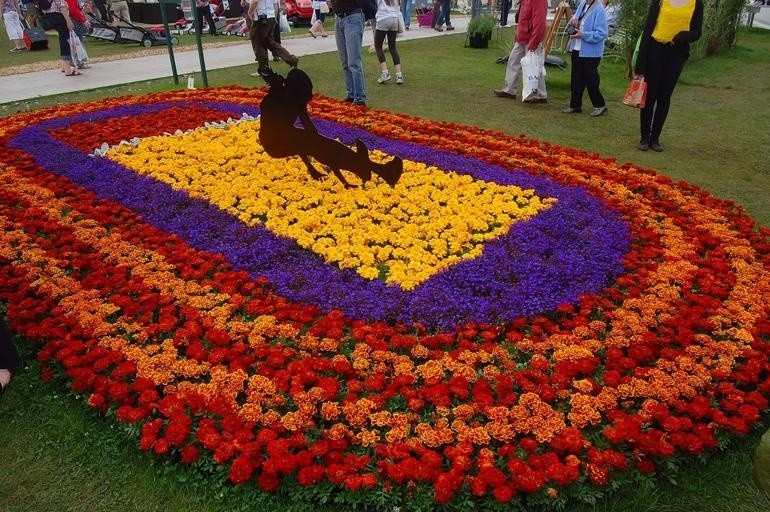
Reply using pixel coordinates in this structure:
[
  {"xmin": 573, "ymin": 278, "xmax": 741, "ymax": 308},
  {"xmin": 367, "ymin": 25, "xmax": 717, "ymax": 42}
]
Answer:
[{"xmin": 23, "ymin": 27, "xmax": 49, "ymax": 51}]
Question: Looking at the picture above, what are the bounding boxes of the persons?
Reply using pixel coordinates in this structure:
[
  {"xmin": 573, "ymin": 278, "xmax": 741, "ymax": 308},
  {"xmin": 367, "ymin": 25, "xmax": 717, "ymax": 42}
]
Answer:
[
  {"xmin": 331, "ymin": 0, "xmax": 377, "ymax": 107},
  {"xmin": 240, "ymin": 0, "xmax": 282, "ymax": 62},
  {"xmin": 0, "ymin": 1, "xmax": 132, "ymax": 77},
  {"xmin": 248, "ymin": 0, "xmax": 298, "ymax": 78},
  {"xmin": 374, "ymin": 0, "xmax": 405, "ymax": 84},
  {"xmin": 398, "ymin": 0, "xmax": 512, "ymax": 31},
  {"xmin": 560, "ymin": 0, "xmax": 609, "ymax": 116},
  {"xmin": 257, "ymin": 65, "xmax": 403, "ymax": 190},
  {"xmin": 309, "ymin": 0, "xmax": 330, "ymax": 38},
  {"xmin": 494, "ymin": 0, "xmax": 548, "ymax": 104},
  {"xmin": 195, "ymin": 0, "xmax": 217, "ymax": 36},
  {"xmin": 635, "ymin": 0, "xmax": 703, "ymax": 152}
]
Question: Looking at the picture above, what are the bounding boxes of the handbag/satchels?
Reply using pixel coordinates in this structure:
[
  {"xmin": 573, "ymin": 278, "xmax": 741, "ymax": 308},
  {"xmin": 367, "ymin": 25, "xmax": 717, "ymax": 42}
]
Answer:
[
  {"xmin": 622, "ymin": 75, "xmax": 648, "ymax": 109},
  {"xmin": 397, "ymin": 8, "xmax": 405, "ymax": 33},
  {"xmin": 319, "ymin": 2, "xmax": 329, "ymax": 13}
]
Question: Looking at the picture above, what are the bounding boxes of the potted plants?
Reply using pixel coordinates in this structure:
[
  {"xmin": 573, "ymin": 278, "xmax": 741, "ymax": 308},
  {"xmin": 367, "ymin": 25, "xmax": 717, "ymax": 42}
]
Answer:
[{"xmin": 467, "ymin": 10, "xmax": 497, "ymax": 50}]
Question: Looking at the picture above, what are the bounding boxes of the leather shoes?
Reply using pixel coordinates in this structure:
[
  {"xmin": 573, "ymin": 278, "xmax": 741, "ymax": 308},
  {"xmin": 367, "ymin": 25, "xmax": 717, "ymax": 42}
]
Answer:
[
  {"xmin": 561, "ymin": 107, "xmax": 582, "ymax": 113},
  {"xmin": 590, "ymin": 106, "xmax": 608, "ymax": 116},
  {"xmin": 494, "ymin": 88, "xmax": 518, "ymax": 99},
  {"xmin": 290, "ymin": 56, "xmax": 299, "ymax": 70},
  {"xmin": 250, "ymin": 68, "xmax": 262, "ymax": 77},
  {"xmin": 651, "ymin": 137, "xmax": 664, "ymax": 152}
]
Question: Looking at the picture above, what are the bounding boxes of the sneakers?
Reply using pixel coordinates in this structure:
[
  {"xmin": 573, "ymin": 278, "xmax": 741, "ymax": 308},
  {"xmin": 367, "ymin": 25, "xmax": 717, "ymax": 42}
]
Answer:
[
  {"xmin": 446, "ymin": 25, "xmax": 454, "ymax": 30},
  {"xmin": 395, "ymin": 73, "xmax": 404, "ymax": 84},
  {"xmin": 342, "ymin": 95, "xmax": 365, "ymax": 107},
  {"xmin": 321, "ymin": 33, "xmax": 328, "ymax": 37},
  {"xmin": 309, "ymin": 28, "xmax": 316, "ymax": 38},
  {"xmin": 435, "ymin": 25, "xmax": 443, "ymax": 32},
  {"xmin": 636, "ymin": 139, "xmax": 650, "ymax": 150},
  {"xmin": 9, "ymin": 45, "xmax": 26, "ymax": 53},
  {"xmin": 376, "ymin": 71, "xmax": 391, "ymax": 84}
]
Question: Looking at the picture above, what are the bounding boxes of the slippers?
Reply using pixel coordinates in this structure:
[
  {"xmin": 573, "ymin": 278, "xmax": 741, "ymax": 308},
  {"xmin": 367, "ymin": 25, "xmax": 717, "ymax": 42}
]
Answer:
[{"xmin": 61, "ymin": 67, "xmax": 82, "ymax": 76}]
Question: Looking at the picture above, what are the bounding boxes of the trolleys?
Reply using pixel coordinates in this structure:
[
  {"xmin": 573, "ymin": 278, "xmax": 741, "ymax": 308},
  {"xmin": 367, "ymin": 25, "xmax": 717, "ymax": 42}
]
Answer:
[{"xmin": 72, "ymin": 0, "xmax": 251, "ymax": 47}]
